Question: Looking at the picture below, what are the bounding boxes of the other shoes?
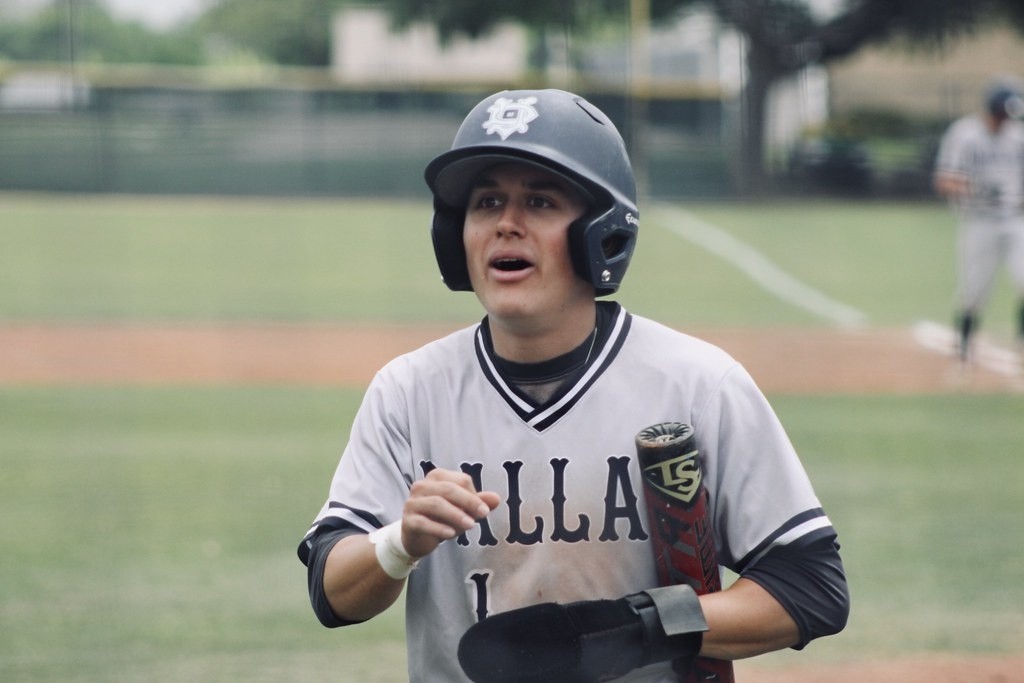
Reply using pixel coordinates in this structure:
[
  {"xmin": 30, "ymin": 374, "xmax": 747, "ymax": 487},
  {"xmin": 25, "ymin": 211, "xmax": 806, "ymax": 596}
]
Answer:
[{"xmin": 954, "ymin": 317, "xmax": 975, "ymax": 360}]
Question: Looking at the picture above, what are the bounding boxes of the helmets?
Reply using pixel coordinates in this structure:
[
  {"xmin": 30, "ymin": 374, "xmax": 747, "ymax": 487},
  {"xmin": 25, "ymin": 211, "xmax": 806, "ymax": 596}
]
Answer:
[
  {"xmin": 425, "ymin": 86, "xmax": 640, "ymax": 297},
  {"xmin": 985, "ymin": 85, "xmax": 1021, "ymax": 116}
]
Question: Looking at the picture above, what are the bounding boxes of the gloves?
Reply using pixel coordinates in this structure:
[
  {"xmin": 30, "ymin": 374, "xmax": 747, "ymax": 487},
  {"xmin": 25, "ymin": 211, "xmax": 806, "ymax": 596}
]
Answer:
[{"xmin": 455, "ymin": 582, "xmax": 709, "ymax": 681}]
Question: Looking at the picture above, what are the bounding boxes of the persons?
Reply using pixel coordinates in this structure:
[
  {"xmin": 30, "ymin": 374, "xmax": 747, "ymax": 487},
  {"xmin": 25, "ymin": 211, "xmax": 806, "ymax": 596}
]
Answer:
[
  {"xmin": 934, "ymin": 83, "xmax": 1023, "ymax": 352},
  {"xmin": 297, "ymin": 86, "xmax": 848, "ymax": 682}
]
